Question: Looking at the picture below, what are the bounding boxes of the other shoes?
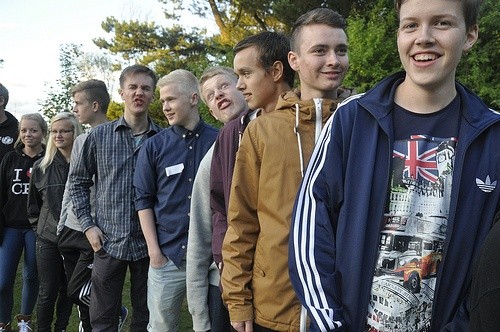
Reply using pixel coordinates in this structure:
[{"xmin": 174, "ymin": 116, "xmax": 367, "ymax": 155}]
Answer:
[
  {"xmin": 15, "ymin": 313, "xmax": 33, "ymax": 332},
  {"xmin": 0, "ymin": 320, "xmax": 10, "ymax": 332},
  {"xmin": 117, "ymin": 307, "xmax": 128, "ymax": 332}
]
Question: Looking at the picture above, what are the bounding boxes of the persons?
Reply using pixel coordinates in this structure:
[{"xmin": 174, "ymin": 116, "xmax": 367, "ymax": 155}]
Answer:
[
  {"xmin": 209, "ymin": 31, "xmax": 295, "ymax": 332},
  {"xmin": 27, "ymin": 111, "xmax": 82, "ymax": 332},
  {"xmin": 69, "ymin": 65, "xmax": 165, "ymax": 332},
  {"xmin": 221, "ymin": 7, "xmax": 357, "ymax": 332},
  {"xmin": 288, "ymin": 0, "xmax": 500, "ymax": 332},
  {"xmin": 133, "ymin": 68, "xmax": 220, "ymax": 332},
  {"xmin": 0, "ymin": 83, "xmax": 20, "ymax": 163},
  {"xmin": 57, "ymin": 79, "xmax": 128, "ymax": 332},
  {"xmin": 0, "ymin": 113, "xmax": 47, "ymax": 332},
  {"xmin": 468, "ymin": 211, "xmax": 500, "ymax": 332},
  {"xmin": 185, "ymin": 66, "xmax": 249, "ymax": 332}
]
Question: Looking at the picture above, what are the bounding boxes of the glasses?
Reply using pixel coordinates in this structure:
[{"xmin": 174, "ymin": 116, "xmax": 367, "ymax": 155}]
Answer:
[{"xmin": 49, "ymin": 130, "xmax": 74, "ymax": 134}]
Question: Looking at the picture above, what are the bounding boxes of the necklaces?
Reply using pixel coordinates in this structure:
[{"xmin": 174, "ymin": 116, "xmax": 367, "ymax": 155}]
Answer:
[{"xmin": 132, "ymin": 130, "xmax": 148, "ymax": 136}]
